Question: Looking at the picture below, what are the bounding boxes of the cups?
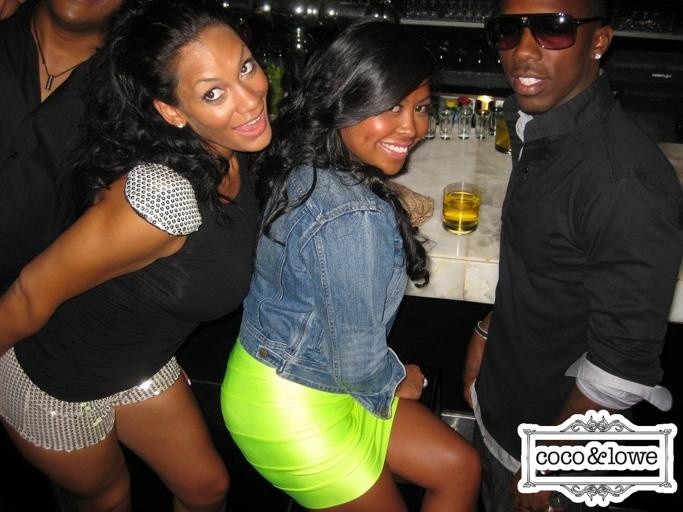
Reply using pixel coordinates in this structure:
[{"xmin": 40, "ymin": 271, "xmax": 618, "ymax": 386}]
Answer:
[
  {"xmin": 438, "ymin": 110, "xmax": 454, "ymax": 140},
  {"xmin": 495, "ymin": 115, "xmax": 511, "ymax": 153},
  {"xmin": 475, "ymin": 109, "xmax": 491, "ymax": 140},
  {"xmin": 457, "ymin": 108, "xmax": 473, "ymax": 140},
  {"xmin": 488, "ymin": 107, "xmax": 504, "ymax": 136},
  {"xmin": 442, "ymin": 182, "xmax": 482, "ymax": 237},
  {"xmin": 425, "ymin": 109, "xmax": 436, "ymax": 139}
]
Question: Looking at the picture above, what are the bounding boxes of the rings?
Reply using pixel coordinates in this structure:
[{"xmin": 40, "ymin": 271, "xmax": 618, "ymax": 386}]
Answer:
[{"xmin": 422, "ymin": 378, "xmax": 428, "ymax": 389}]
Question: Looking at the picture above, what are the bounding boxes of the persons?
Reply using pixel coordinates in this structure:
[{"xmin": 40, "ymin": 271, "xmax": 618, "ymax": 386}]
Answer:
[
  {"xmin": 0, "ymin": 0, "xmax": 146, "ymax": 302},
  {"xmin": 1, "ymin": 1, "xmax": 274, "ymax": 512},
  {"xmin": 458, "ymin": 0, "xmax": 681, "ymax": 512},
  {"xmin": 218, "ymin": 14, "xmax": 482, "ymax": 511}
]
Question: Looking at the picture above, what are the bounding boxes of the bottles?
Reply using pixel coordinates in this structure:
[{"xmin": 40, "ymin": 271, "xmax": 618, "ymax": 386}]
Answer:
[{"xmin": 402, "ymin": 1, "xmax": 494, "ymax": 22}]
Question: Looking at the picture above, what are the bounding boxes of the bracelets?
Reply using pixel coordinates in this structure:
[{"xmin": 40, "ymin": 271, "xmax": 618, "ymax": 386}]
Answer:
[{"xmin": 474, "ymin": 321, "xmax": 488, "ymax": 340}]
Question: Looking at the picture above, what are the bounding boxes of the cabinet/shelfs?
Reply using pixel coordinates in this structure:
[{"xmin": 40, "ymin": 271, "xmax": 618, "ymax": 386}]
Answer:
[{"xmin": 238, "ymin": 3, "xmax": 683, "ymax": 94}]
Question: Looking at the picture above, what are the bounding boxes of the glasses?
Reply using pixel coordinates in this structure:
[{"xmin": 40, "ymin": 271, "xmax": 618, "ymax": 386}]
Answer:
[{"xmin": 484, "ymin": 11, "xmax": 603, "ymax": 50}]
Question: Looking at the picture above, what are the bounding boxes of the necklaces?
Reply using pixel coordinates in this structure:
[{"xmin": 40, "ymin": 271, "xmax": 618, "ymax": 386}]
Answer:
[{"xmin": 31, "ymin": 5, "xmax": 85, "ymax": 92}]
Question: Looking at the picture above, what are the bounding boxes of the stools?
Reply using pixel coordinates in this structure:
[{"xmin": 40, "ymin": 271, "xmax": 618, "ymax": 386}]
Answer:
[{"xmin": 286, "ymin": 366, "xmax": 446, "ymax": 512}]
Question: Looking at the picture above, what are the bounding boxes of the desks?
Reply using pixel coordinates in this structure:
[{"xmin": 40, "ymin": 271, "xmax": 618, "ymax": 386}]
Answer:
[{"xmin": 401, "ymin": 116, "xmax": 683, "ymax": 324}]
